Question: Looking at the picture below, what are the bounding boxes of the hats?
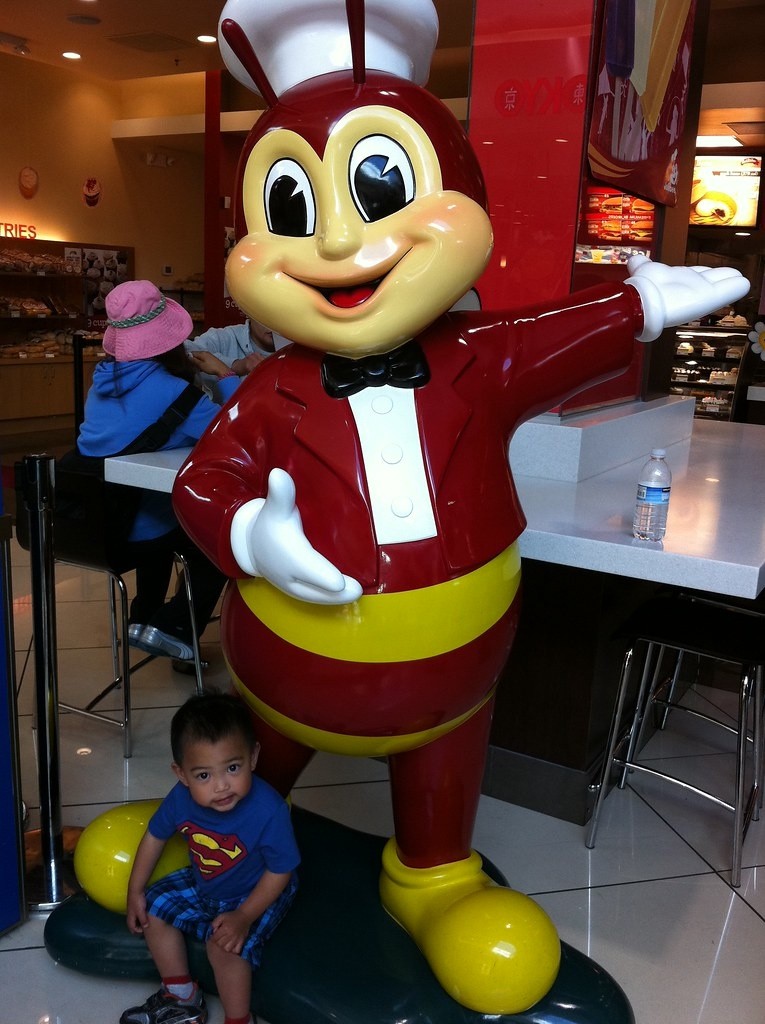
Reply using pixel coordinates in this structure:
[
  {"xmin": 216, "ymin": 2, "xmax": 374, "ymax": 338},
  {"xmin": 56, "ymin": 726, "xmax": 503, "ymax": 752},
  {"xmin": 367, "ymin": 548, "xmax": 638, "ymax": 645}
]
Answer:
[{"xmin": 102, "ymin": 280, "xmax": 193, "ymax": 361}]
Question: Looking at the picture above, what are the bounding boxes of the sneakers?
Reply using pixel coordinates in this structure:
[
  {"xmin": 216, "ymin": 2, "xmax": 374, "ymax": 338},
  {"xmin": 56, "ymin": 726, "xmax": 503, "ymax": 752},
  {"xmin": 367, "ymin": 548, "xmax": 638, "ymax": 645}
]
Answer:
[{"xmin": 119, "ymin": 975, "xmax": 208, "ymax": 1024}]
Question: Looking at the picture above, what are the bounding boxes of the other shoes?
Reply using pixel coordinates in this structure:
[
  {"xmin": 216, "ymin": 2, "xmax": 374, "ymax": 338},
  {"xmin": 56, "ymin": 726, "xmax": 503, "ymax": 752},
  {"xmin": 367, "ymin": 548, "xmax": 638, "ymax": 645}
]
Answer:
[{"xmin": 128, "ymin": 623, "xmax": 194, "ymax": 661}]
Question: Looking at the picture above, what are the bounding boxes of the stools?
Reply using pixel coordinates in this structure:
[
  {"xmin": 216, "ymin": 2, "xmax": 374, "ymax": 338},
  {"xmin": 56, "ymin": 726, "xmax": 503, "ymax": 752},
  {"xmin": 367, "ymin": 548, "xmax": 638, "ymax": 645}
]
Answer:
[
  {"xmin": 661, "ymin": 595, "xmax": 764, "ymax": 733},
  {"xmin": 588, "ymin": 613, "xmax": 761, "ymax": 894},
  {"xmin": 15, "ymin": 451, "xmax": 207, "ymax": 755}
]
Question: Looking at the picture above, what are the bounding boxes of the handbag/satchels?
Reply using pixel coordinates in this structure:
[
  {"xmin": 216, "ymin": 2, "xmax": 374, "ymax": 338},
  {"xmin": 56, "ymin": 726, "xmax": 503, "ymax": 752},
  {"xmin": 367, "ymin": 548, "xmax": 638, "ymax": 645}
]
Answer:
[{"xmin": 55, "ymin": 382, "xmax": 206, "ymax": 546}]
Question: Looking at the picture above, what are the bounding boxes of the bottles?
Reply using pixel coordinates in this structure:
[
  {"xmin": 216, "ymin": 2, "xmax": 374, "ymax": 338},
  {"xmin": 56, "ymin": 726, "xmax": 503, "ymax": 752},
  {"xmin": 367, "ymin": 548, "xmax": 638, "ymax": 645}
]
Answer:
[{"xmin": 632, "ymin": 448, "xmax": 671, "ymax": 542}]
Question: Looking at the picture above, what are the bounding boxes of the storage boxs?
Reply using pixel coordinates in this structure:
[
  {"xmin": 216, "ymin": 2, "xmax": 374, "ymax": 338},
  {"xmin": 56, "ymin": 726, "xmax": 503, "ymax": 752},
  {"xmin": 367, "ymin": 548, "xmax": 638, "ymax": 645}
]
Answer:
[{"xmin": 505, "ymin": 395, "xmax": 696, "ymax": 481}]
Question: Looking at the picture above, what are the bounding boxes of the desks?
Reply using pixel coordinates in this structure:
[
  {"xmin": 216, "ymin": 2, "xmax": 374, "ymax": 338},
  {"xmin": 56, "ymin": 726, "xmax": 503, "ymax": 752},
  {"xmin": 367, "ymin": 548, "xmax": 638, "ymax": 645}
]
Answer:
[{"xmin": 105, "ymin": 418, "xmax": 765, "ymax": 829}]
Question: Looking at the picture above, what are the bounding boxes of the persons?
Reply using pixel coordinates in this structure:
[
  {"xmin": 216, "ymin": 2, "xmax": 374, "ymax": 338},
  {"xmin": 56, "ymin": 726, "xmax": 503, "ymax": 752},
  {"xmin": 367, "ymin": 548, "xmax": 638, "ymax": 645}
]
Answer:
[
  {"xmin": 76, "ymin": 279, "xmax": 278, "ymax": 662},
  {"xmin": 119, "ymin": 685, "xmax": 308, "ymax": 1024}
]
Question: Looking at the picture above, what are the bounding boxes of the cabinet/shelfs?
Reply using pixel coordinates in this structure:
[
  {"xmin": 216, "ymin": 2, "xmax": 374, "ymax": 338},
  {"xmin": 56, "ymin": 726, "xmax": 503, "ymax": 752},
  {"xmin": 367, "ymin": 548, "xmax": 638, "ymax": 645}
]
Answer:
[
  {"xmin": 0, "ymin": 236, "xmax": 135, "ymax": 418},
  {"xmin": 648, "ymin": 324, "xmax": 752, "ymax": 421}
]
